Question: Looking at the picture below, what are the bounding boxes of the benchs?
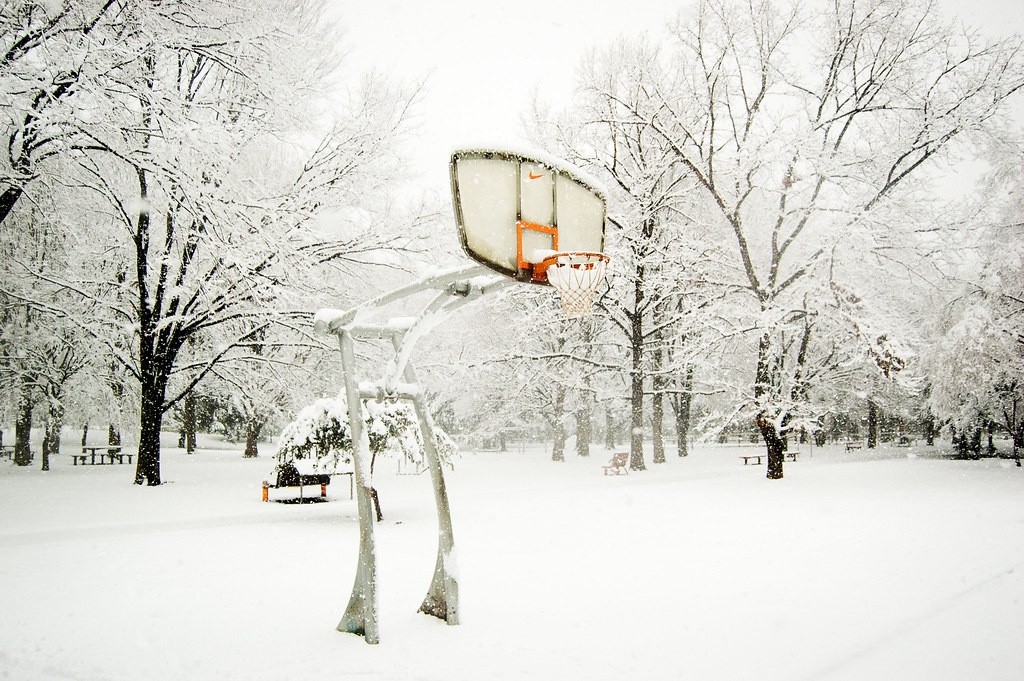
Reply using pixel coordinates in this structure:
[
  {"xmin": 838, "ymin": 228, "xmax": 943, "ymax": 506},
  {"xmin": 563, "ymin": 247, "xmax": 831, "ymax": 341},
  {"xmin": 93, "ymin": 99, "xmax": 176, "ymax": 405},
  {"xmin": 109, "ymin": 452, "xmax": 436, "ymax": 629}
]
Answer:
[
  {"xmin": 72, "ymin": 453, "xmax": 132, "ymax": 465},
  {"xmin": 602, "ymin": 453, "xmax": 629, "ymax": 476},
  {"xmin": 844, "ymin": 442, "xmax": 864, "ymax": 453},
  {"xmin": 740, "ymin": 452, "xmax": 801, "ymax": 465},
  {"xmin": 7, "ymin": 451, "xmax": 37, "ymax": 459}
]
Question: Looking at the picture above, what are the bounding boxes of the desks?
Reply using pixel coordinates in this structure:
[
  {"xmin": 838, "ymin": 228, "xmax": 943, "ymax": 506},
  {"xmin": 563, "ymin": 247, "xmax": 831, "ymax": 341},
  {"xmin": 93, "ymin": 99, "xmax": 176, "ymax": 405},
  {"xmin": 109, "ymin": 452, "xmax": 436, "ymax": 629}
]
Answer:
[
  {"xmin": 80, "ymin": 447, "xmax": 122, "ymax": 465},
  {"xmin": 0, "ymin": 445, "xmax": 15, "ymax": 458}
]
